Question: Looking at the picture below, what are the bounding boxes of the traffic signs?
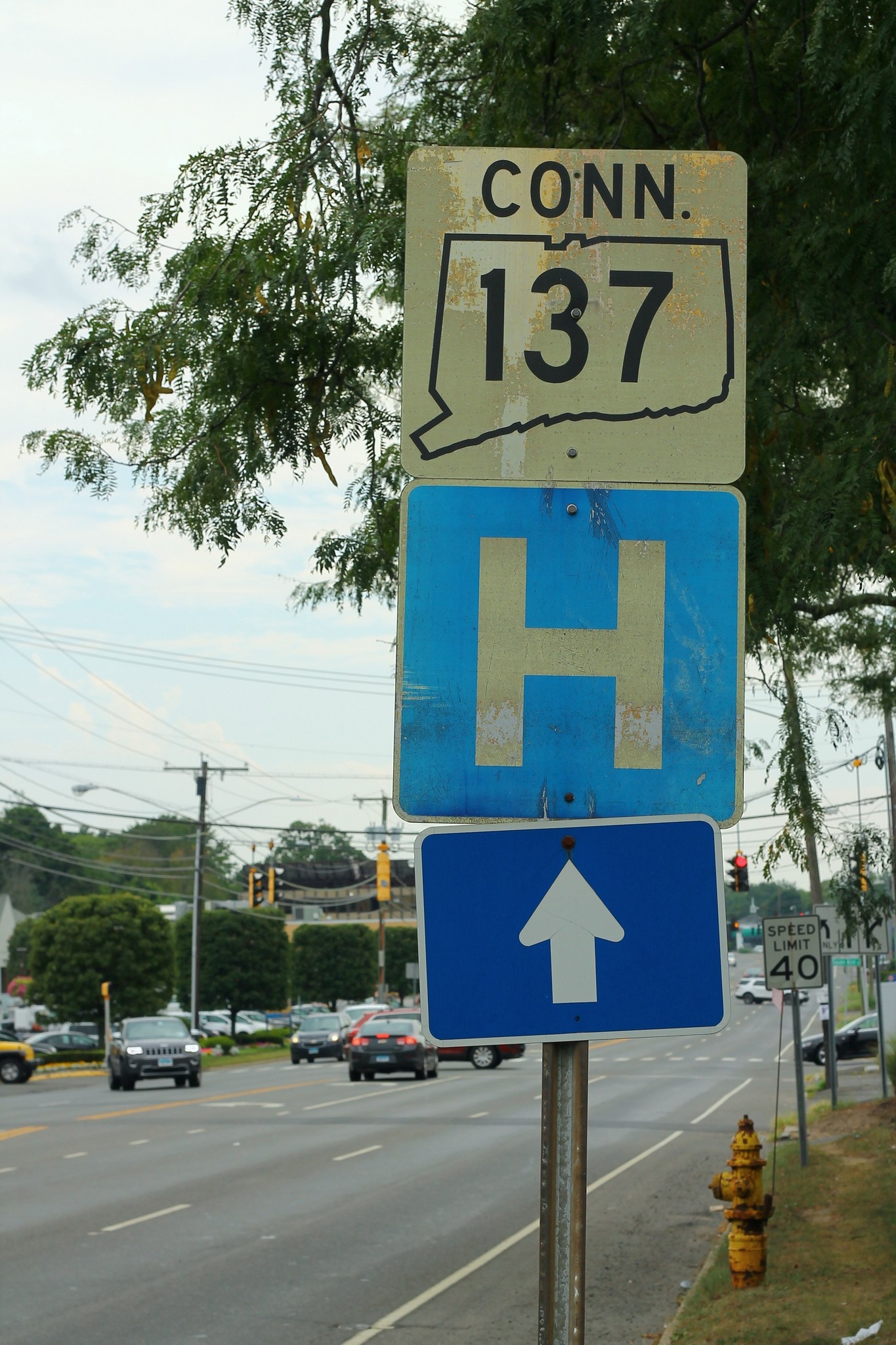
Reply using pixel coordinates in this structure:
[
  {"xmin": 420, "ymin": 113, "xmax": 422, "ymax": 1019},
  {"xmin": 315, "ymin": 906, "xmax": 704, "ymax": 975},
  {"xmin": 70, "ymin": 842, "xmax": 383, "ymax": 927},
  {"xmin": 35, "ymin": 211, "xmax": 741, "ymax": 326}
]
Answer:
[{"xmin": 812, "ymin": 903, "xmax": 892, "ymax": 955}]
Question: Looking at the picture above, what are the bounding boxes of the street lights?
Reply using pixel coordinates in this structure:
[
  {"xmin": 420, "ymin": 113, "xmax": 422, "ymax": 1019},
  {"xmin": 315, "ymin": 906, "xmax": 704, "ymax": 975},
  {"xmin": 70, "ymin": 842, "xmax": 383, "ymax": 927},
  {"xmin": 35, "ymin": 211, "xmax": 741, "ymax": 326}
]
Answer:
[{"xmin": 70, "ymin": 785, "xmax": 204, "ymax": 1040}]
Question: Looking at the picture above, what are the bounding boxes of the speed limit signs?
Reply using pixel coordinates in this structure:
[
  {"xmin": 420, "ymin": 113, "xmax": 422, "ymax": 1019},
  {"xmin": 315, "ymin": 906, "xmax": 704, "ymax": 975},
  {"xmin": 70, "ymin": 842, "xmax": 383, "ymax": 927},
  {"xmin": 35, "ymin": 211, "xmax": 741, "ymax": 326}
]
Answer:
[{"xmin": 762, "ymin": 915, "xmax": 822, "ymax": 990}]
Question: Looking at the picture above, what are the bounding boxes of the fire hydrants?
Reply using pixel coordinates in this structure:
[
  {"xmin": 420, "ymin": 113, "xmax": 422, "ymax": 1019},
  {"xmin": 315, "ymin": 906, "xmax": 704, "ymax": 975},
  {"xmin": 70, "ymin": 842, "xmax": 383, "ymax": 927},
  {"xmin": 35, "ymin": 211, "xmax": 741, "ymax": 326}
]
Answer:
[{"xmin": 708, "ymin": 1115, "xmax": 777, "ymax": 1291}]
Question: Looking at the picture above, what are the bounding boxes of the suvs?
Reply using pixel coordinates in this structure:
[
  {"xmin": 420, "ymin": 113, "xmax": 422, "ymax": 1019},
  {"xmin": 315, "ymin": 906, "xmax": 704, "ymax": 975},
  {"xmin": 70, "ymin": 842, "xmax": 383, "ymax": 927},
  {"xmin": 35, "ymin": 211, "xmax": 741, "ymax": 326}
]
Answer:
[
  {"xmin": 735, "ymin": 978, "xmax": 809, "ymax": 1006},
  {"xmin": 343, "ymin": 1008, "xmax": 526, "ymax": 1071},
  {"xmin": 109, "ymin": 1016, "xmax": 203, "ymax": 1091}
]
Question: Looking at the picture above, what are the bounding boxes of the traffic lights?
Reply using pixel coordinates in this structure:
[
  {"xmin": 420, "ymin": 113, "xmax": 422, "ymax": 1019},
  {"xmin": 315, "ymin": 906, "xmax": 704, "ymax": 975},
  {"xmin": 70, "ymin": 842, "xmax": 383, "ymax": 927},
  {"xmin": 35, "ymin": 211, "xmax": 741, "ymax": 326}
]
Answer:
[
  {"xmin": 731, "ymin": 917, "xmax": 739, "ymax": 931},
  {"xmin": 100, "ymin": 982, "xmax": 114, "ymax": 996},
  {"xmin": 726, "ymin": 855, "xmax": 750, "ymax": 892}
]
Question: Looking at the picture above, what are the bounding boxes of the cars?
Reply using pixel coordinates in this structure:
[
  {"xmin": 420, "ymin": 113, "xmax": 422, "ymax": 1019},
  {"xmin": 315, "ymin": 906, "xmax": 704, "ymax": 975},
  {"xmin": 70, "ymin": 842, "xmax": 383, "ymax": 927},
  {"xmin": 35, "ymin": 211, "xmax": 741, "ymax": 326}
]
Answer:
[
  {"xmin": 753, "ymin": 945, "xmax": 764, "ymax": 953},
  {"xmin": 744, "ymin": 968, "xmax": 763, "ymax": 978},
  {"xmin": 0, "ymin": 1023, "xmax": 100, "ymax": 1085},
  {"xmin": 727, "ymin": 952, "xmax": 737, "ymax": 966},
  {"xmin": 170, "ymin": 998, "xmax": 401, "ymax": 1065},
  {"xmin": 349, "ymin": 1019, "xmax": 438, "ymax": 1081},
  {"xmin": 802, "ymin": 1013, "xmax": 879, "ymax": 1065}
]
